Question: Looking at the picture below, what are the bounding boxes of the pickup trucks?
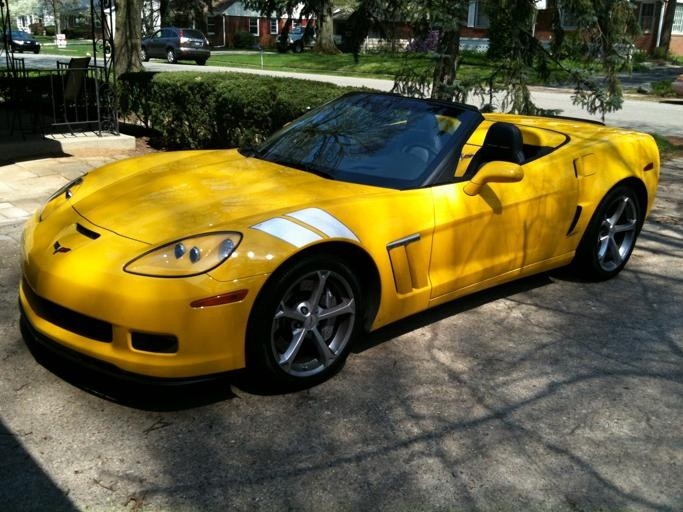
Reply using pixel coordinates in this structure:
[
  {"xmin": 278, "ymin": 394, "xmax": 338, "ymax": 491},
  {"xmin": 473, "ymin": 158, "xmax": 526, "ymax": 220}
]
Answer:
[{"xmin": 275, "ymin": 26, "xmax": 341, "ymax": 53}]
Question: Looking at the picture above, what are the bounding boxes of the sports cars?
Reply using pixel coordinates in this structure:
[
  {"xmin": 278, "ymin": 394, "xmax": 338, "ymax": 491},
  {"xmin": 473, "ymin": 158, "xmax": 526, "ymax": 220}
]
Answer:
[{"xmin": 18, "ymin": 90, "xmax": 661, "ymax": 386}]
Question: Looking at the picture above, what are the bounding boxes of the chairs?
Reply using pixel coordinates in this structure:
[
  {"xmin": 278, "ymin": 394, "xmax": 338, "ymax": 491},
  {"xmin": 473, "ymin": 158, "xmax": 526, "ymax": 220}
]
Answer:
[
  {"xmin": 463, "ymin": 121, "xmax": 527, "ymax": 179},
  {"xmin": 383, "ymin": 110, "xmax": 446, "ymax": 163},
  {"xmin": 1, "ymin": 57, "xmax": 90, "ymax": 140}
]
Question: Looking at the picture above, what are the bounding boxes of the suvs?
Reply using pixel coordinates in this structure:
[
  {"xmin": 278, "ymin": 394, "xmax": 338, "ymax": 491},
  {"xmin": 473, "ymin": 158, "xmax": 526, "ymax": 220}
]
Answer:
[
  {"xmin": 140, "ymin": 27, "xmax": 210, "ymax": 65},
  {"xmin": 6, "ymin": 31, "xmax": 39, "ymax": 54}
]
